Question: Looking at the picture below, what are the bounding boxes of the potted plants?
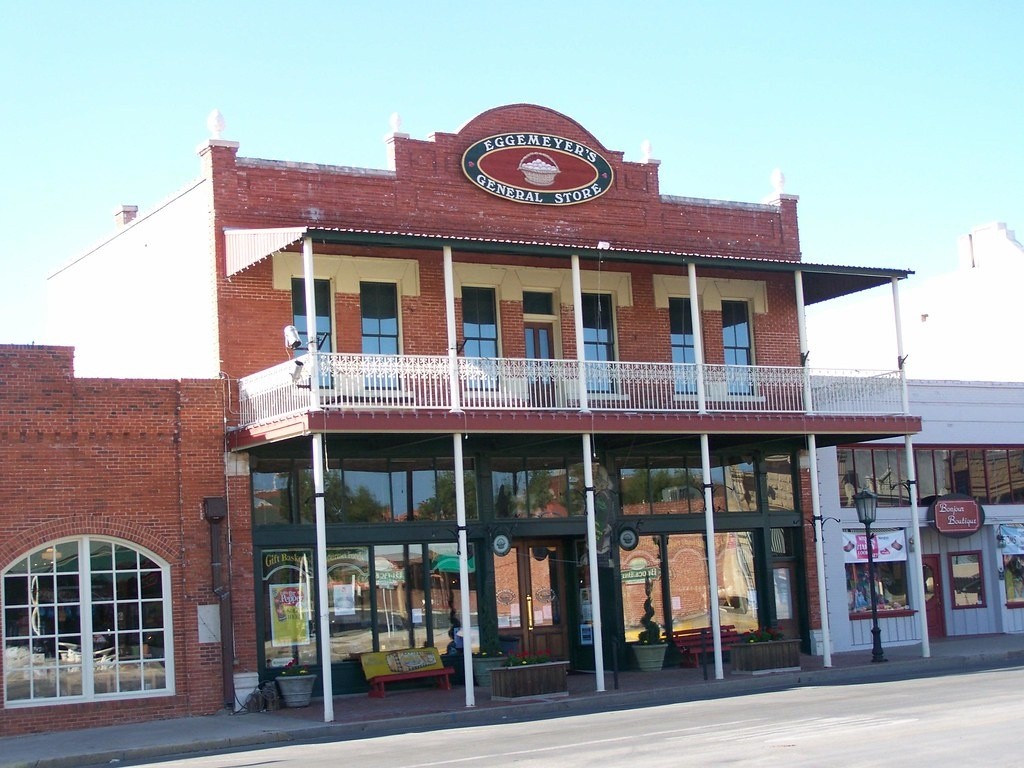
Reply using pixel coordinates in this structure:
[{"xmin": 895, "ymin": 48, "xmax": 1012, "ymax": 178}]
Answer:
[{"xmin": 631, "ymin": 573, "xmax": 668, "ymax": 672}]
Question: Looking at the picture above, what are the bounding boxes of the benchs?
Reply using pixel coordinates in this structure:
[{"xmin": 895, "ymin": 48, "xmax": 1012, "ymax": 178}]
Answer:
[
  {"xmin": 360, "ymin": 647, "xmax": 456, "ymax": 698},
  {"xmin": 670, "ymin": 625, "xmax": 740, "ymax": 669}
]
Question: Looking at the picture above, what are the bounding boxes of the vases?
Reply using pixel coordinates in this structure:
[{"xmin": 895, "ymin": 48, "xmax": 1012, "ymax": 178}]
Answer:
[
  {"xmin": 473, "ymin": 657, "xmax": 508, "ymax": 687},
  {"xmin": 275, "ymin": 674, "xmax": 317, "ymax": 709},
  {"xmin": 439, "ymin": 655, "xmax": 465, "ymax": 687}
]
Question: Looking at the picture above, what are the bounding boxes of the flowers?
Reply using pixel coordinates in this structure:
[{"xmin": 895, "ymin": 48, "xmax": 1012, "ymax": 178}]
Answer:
[
  {"xmin": 280, "ymin": 658, "xmax": 310, "ymax": 677},
  {"xmin": 741, "ymin": 624, "xmax": 785, "ymax": 643},
  {"xmin": 472, "ymin": 577, "xmax": 506, "ymax": 658},
  {"xmin": 499, "ymin": 644, "xmax": 554, "ymax": 667}
]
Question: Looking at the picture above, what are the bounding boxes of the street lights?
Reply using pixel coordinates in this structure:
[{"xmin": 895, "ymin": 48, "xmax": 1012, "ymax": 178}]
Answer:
[{"xmin": 850, "ymin": 488, "xmax": 888, "ymax": 664}]
